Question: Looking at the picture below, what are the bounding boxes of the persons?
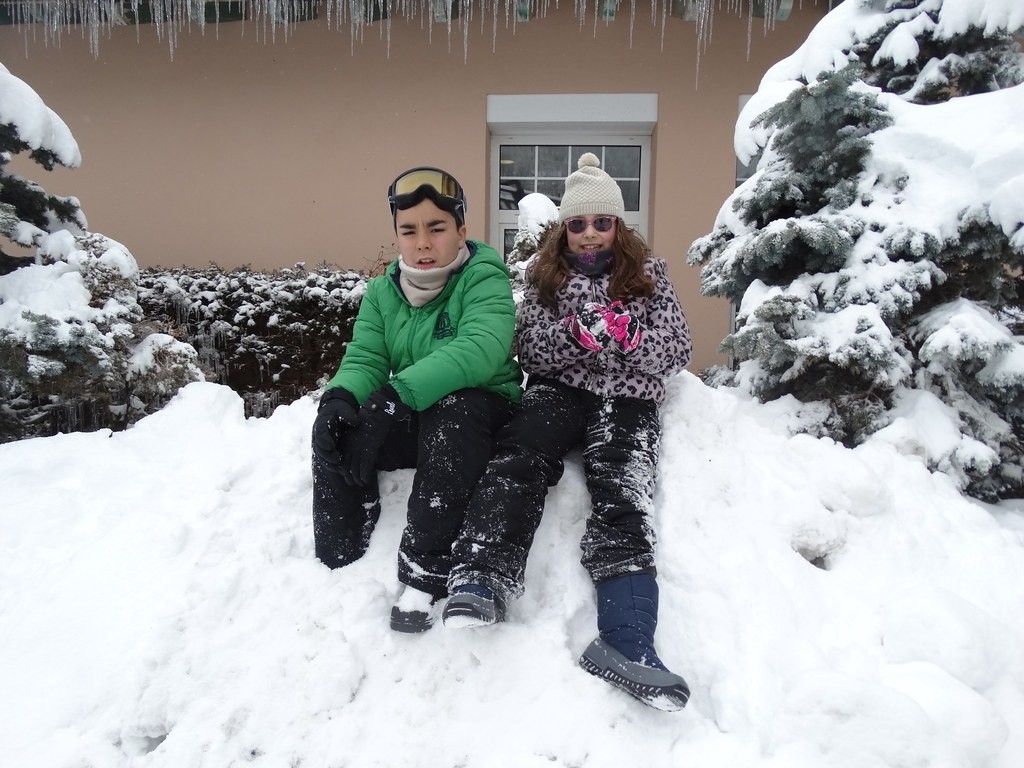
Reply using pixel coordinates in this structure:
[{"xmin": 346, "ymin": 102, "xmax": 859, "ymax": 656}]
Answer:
[
  {"xmin": 312, "ymin": 166, "xmax": 525, "ymax": 634},
  {"xmin": 441, "ymin": 152, "xmax": 691, "ymax": 713}
]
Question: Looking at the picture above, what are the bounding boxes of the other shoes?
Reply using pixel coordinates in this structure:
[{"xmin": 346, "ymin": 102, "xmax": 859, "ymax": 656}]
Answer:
[{"xmin": 389, "ymin": 578, "xmax": 442, "ymax": 633}]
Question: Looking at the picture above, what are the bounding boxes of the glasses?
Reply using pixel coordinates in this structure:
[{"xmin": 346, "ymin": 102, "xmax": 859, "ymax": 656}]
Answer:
[
  {"xmin": 386, "ymin": 168, "xmax": 464, "ymax": 226},
  {"xmin": 562, "ymin": 215, "xmax": 615, "ymax": 233}
]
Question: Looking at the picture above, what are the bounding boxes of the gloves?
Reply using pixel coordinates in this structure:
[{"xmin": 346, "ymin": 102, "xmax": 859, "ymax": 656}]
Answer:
[
  {"xmin": 311, "ymin": 387, "xmax": 360, "ymax": 471},
  {"xmin": 566, "ymin": 298, "xmax": 620, "ymax": 353},
  {"xmin": 359, "ymin": 382, "xmax": 414, "ymax": 446},
  {"xmin": 611, "ymin": 301, "xmax": 643, "ymax": 357}
]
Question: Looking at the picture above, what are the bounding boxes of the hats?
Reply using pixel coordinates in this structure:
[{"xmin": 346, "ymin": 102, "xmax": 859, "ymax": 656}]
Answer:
[{"xmin": 557, "ymin": 152, "xmax": 625, "ymax": 222}]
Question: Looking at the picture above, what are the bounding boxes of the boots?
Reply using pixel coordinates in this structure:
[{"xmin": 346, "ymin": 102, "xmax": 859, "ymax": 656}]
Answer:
[
  {"xmin": 580, "ymin": 568, "xmax": 689, "ymax": 713},
  {"xmin": 441, "ymin": 583, "xmax": 509, "ymax": 626}
]
